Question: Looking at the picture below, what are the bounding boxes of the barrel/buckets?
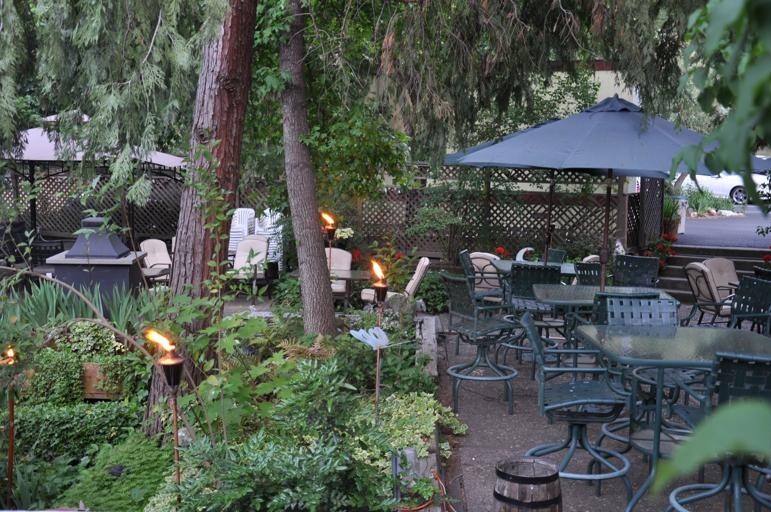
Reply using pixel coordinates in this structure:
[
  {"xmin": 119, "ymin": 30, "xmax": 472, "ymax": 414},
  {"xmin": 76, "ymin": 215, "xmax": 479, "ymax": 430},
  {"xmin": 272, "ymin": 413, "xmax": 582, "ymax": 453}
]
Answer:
[
  {"xmin": 82, "ymin": 360, "xmax": 123, "ymax": 401},
  {"xmin": 492, "ymin": 457, "xmax": 563, "ymax": 511}
]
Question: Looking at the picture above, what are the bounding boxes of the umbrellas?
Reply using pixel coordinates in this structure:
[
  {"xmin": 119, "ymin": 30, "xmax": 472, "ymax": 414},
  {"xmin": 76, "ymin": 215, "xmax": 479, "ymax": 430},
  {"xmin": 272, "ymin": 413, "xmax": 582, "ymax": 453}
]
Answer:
[
  {"xmin": 442, "ymin": 93, "xmax": 770, "ymax": 291},
  {"xmin": 0, "ymin": 106, "xmax": 189, "ymax": 266}
]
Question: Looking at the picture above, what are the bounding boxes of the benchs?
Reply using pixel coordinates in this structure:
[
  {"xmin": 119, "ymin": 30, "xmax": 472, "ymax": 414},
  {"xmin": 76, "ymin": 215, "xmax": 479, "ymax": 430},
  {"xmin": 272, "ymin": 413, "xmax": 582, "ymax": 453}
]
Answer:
[{"xmin": 25, "ymin": 239, "xmax": 65, "ymax": 272}]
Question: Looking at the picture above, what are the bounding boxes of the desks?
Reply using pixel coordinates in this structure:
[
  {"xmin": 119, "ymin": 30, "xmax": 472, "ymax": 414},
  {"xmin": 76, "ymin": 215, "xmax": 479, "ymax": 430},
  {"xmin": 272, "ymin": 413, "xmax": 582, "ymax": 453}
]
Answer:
[
  {"xmin": 329, "ymin": 269, "xmax": 371, "ymax": 309},
  {"xmin": 490, "ymin": 258, "xmax": 579, "ymax": 293},
  {"xmin": 532, "ymin": 283, "xmax": 662, "ymax": 374},
  {"xmin": 578, "ymin": 322, "xmax": 771, "ymax": 509}
]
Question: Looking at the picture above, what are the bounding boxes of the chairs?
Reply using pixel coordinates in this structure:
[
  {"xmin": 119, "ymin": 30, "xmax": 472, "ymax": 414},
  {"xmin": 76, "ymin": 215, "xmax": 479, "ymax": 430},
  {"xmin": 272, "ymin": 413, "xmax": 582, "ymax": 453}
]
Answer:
[
  {"xmin": 726, "ymin": 276, "xmax": 771, "ymax": 336},
  {"xmin": 703, "ymin": 258, "xmax": 741, "ymax": 309},
  {"xmin": 138, "ymin": 235, "xmax": 176, "ymax": 300},
  {"xmin": 683, "ymin": 263, "xmax": 732, "ymax": 328},
  {"xmin": 753, "ymin": 265, "xmax": 771, "ymax": 280},
  {"xmin": 542, "ymin": 248, "xmax": 566, "ymax": 261},
  {"xmin": 666, "ymin": 312, "xmax": 771, "ymax": 427},
  {"xmin": 509, "ymin": 262, "xmax": 562, "ymax": 298},
  {"xmin": 667, "ymin": 353, "xmax": 770, "ymax": 512},
  {"xmin": 361, "ymin": 255, "xmax": 430, "ymax": 306},
  {"xmin": 615, "ymin": 254, "xmax": 660, "ymax": 287},
  {"xmin": 323, "ymin": 247, "xmax": 353, "ymax": 315},
  {"xmin": 458, "ymin": 249, "xmax": 508, "ymax": 305},
  {"xmin": 224, "ymin": 208, "xmax": 286, "ymax": 300},
  {"xmin": 440, "ymin": 270, "xmax": 518, "ymax": 418},
  {"xmin": 573, "ymin": 261, "xmax": 608, "ymax": 286},
  {"xmin": 520, "ymin": 310, "xmax": 634, "ymax": 503},
  {"xmin": 600, "ymin": 298, "xmax": 681, "ymax": 453}
]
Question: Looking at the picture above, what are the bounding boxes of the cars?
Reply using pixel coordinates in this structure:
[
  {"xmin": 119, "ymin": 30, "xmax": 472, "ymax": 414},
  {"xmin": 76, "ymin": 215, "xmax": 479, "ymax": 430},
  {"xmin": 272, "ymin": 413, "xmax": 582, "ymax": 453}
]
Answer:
[{"xmin": 637, "ymin": 152, "xmax": 771, "ymax": 205}]
